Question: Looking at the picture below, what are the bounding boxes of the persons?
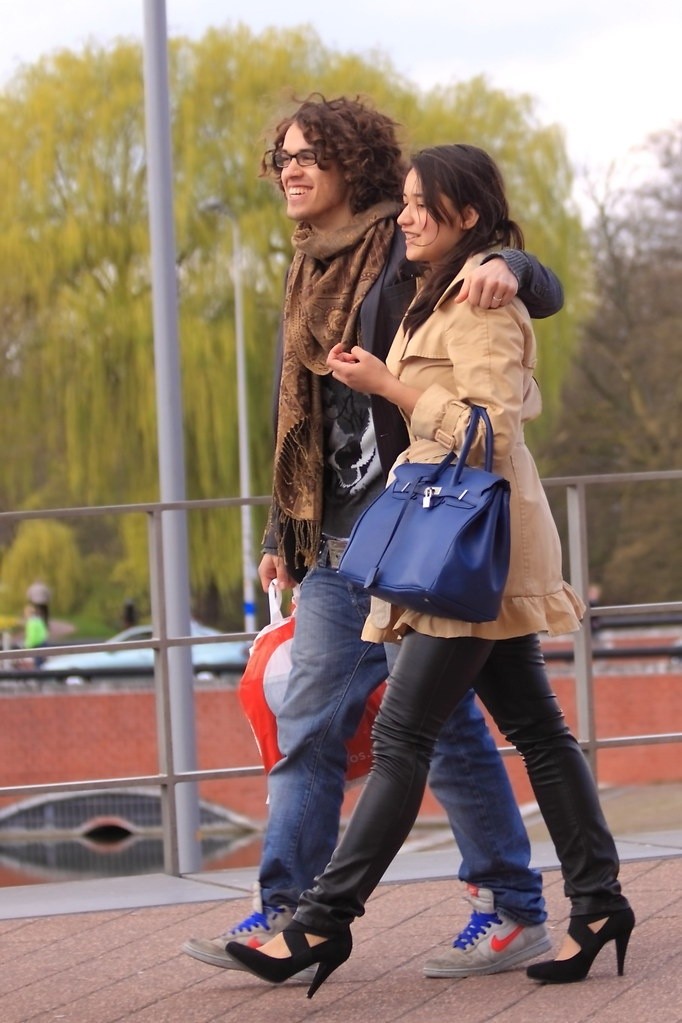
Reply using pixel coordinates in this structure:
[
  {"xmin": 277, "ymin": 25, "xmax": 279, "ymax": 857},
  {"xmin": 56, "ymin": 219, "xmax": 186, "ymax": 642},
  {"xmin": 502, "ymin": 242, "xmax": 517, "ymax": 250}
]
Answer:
[
  {"xmin": 589, "ymin": 584, "xmax": 610, "ymax": 637},
  {"xmin": 178, "ymin": 97, "xmax": 553, "ymax": 978},
  {"xmin": 227, "ymin": 142, "xmax": 637, "ymax": 1000},
  {"xmin": 21, "ymin": 580, "xmax": 52, "ymax": 671}
]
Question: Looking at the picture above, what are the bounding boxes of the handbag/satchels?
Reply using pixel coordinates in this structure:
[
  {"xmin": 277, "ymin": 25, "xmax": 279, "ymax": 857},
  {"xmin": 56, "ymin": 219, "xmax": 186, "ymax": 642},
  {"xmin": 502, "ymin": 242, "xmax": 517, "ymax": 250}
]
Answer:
[
  {"xmin": 239, "ymin": 577, "xmax": 395, "ymax": 805},
  {"xmin": 337, "ymin": 408, "xmax": 512, "ymax": 624}
]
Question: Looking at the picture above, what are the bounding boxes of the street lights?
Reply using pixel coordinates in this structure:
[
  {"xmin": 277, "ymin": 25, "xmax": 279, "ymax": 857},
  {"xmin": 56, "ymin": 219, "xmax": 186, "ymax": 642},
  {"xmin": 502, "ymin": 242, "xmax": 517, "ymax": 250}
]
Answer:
[{"xmin": 196, "ymin": 197, "xmax": 258, "ymax": 631}]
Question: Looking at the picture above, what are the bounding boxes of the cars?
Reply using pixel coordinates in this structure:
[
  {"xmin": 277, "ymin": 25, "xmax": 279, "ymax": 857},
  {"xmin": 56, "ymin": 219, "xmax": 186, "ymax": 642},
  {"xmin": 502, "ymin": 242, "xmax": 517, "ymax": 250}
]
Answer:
[{"xmin": 40, "ymin": 620, "xmax": 254, "ymax": 686}]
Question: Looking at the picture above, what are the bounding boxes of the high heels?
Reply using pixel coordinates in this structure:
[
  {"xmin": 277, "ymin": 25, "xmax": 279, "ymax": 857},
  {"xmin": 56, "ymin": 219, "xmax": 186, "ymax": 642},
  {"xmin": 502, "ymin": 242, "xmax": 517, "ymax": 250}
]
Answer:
[
  {"xmin": 225, "ymin": 926, "xmax": 353, "ymax": 1000},
  {"xmin": 527, "ymin": 908, "xmax": 635, "ymax": 984}
]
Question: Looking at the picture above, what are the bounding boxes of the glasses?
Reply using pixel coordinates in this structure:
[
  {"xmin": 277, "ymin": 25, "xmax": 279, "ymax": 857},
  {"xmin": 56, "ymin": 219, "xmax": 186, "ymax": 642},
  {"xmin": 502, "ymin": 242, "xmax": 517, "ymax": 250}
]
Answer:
[{"xmin": 275, "ymin": 149, "xmax": 329, "ymax": 168}]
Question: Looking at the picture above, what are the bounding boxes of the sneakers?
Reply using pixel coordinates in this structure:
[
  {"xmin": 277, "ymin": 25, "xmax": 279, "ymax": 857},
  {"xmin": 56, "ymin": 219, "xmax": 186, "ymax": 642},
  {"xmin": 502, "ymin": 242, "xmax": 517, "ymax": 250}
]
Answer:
[
  {"xmin": 421, "ymin": 882, "xmax": 553, "ymax": 978},
  {"xmin": 184, "ymin": 904, "xmax": 321, "ymax": 982}
]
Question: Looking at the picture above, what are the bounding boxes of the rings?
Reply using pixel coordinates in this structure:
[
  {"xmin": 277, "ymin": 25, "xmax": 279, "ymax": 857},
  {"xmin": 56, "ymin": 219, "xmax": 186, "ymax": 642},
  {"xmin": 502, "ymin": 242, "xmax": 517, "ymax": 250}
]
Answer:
[{"xmin": 493, "ymin": 297, "xmax": 502, "ymax": 302}]
